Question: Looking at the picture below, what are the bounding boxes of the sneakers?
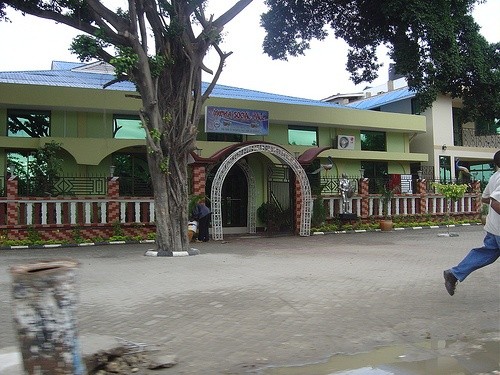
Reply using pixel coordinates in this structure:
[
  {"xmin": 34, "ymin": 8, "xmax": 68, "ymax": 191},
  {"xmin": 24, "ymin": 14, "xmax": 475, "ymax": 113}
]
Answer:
[{"xmin": 443, "ymin": 269, "xmax": 457, "ymax": 296}]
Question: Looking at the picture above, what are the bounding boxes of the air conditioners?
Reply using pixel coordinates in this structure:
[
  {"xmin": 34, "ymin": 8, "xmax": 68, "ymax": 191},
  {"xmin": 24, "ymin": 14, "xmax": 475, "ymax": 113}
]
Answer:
[{"xmin": 336, "ymin": 134, "xmax": 355, "ymax": 149}]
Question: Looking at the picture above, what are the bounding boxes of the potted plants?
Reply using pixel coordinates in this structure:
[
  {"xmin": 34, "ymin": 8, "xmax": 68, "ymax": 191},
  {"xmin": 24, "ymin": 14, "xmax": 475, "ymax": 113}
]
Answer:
[
  {"xmin": 188, "ymin": 193, "xmax": 205, "ymax": 243},
  {"xmin": 379, "ymin": 184, "xmax": 398, "ymax": 232},
  {"xmin": 480, "ymin": 204, "xmax": 488, "ymax": 225}
]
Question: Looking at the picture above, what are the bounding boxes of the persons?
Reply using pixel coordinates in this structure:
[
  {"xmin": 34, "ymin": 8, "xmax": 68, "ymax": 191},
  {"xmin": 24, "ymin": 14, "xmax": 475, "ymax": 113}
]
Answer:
[
  {"xmin": 193, "ymin": 204, "xmax": 212, "ymax": 243},
  {"xmin": 339, "ymin": 172, "xmax": 352, "ymax": 214},
  {"xmin": 443, "ymin": 149, "xmax": 500, "ymax": 298}
]
